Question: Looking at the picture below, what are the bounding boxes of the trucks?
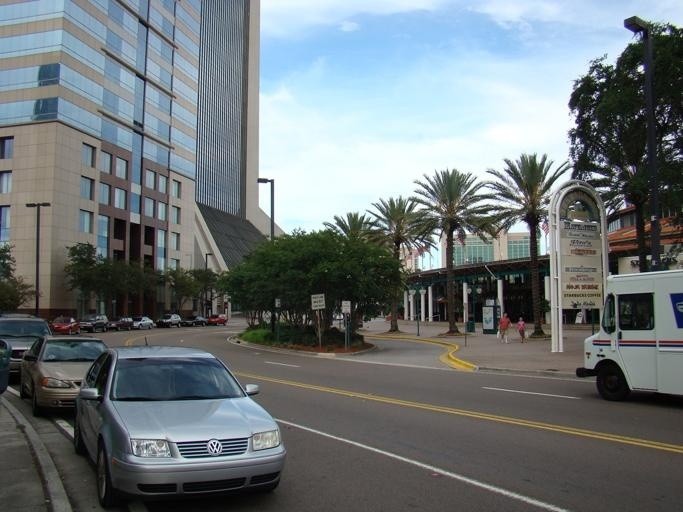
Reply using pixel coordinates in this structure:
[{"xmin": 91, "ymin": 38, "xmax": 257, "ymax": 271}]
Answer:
[{"xmin": 575, "ymin": 265, "xmax": 682, "ymax": 407}]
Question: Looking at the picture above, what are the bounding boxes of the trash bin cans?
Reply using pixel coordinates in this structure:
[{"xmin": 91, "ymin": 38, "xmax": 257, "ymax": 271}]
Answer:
[{"xmin": 466, "ymin": 320, "xmax": 476, "ymax": 333}]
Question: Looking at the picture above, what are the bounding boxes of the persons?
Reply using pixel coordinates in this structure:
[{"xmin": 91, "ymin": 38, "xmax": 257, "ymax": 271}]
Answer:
[
  {"xmin": 497, "ymin": 312, "xmax": 512, "ymax": 344},
  {"xmin": 514, "ymin": 316, "xmax": 527, "ymax": 344}
]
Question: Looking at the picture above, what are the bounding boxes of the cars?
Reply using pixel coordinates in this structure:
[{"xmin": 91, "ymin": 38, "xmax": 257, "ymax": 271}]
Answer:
[
  {"xmin": 131, "ymin": 316, "xmax": 153, "ymax": 330},
  {"xmin": 180, "ymin": 313, "xmax": 227, "ymax": 327},
  {"xmin": 22, "ymin": 335, "xmax": 109, "ymax": 417},
  {"xmin": 74, "ymin": 345, "xmax": 286, "ymax": 510},
  {"xmin": 50, "ymin": 316, "xmax": 81, "ymax": 336}
]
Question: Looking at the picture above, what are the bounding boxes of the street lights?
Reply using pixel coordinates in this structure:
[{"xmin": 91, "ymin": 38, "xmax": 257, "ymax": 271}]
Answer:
[
  {"xmin": 465, "ymin": 279, "xmax": 481, "ymax": 333},
  {"xmin": 254, "ymin": 175, "xmax": 278, "ymax": 342},
  {"xmin": 21, "ymin": 200, "xmax": 51, "ymax": 318},
  {"xmin": 201, "ymin": 252, "xmax": 213, "ymax": 318},
  {"xmin": 405, "ymin": 280, "xmax": 428, "ymax": 337}
]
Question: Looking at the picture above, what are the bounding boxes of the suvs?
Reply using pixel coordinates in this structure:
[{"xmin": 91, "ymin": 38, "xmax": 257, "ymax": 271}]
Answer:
[
  {"xmin": 78, "ymin": 314, "xmax": 109, "ymax": 333},
  {"xmin": 155, "ymin": 314, "xmax": 182, "ymax": 328},
  {"xmin": 0, "ymin": 313, "xmax": 53, "ymax": 378},
  {"xmin": 106, "ymin": 316, "xmax": 134, "ymax": 331}
]
